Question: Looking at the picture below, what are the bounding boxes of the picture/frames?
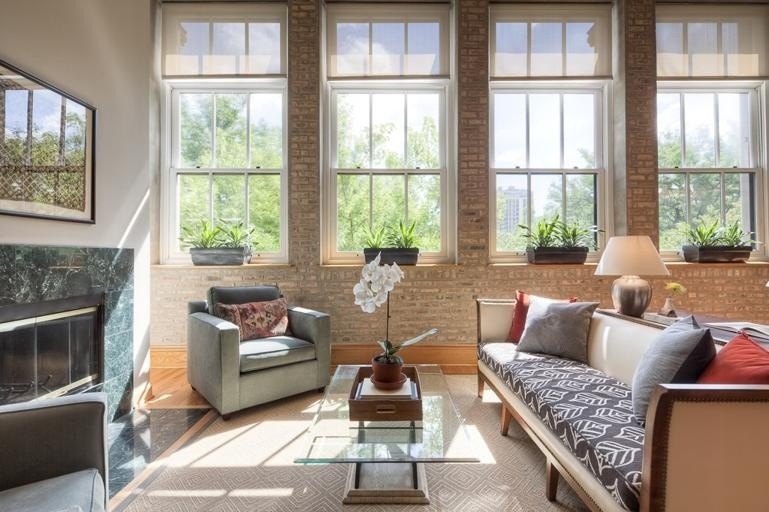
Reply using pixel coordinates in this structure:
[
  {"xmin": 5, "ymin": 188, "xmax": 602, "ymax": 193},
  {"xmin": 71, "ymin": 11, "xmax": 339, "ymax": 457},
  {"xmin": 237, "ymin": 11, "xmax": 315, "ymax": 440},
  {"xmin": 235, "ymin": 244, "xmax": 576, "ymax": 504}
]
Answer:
[{"xmin": 0, "ymin": 59, "xmax": 97, "ymax": 226}]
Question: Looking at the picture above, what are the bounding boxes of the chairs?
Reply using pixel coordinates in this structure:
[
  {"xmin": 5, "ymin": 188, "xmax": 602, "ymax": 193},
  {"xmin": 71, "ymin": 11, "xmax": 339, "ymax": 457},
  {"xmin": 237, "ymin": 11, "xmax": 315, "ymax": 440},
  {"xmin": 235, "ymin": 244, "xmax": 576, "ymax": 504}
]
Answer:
[
  {"xmin": 0, "ymin": 393, "xmax": 110, "ymax": 512},
  {"xmin": 187, "ymin": 284, "xmax": 332, "ymax": 419}
]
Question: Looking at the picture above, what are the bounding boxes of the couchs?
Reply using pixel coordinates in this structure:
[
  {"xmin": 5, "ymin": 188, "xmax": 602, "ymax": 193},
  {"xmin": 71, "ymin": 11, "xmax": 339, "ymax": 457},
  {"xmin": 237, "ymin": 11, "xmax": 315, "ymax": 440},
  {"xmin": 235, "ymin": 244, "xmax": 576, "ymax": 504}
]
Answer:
[{"xmin": 468, "ymin": 293, "xmax": 769, "ymax": 512}]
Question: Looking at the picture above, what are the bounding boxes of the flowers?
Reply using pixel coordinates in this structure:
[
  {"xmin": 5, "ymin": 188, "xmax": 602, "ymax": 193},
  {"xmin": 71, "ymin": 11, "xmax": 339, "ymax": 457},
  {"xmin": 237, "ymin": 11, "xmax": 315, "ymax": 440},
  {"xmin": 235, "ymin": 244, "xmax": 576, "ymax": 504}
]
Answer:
[
  {"xmin": 352, "ymin": 250, "xmax": 437, "ymax": 364},
  {"xmin": 663, "ymin": 282, "xmax": 688, "ymax": 298}
]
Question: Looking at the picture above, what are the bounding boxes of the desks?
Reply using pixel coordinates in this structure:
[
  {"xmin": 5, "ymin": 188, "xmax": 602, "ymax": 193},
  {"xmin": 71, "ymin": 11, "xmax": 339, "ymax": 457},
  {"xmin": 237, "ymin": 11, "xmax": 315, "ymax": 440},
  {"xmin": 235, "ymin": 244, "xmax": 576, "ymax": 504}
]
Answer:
[{"xmin": 592, "ymin": 304, "xmax": 768, "ymax": 366}]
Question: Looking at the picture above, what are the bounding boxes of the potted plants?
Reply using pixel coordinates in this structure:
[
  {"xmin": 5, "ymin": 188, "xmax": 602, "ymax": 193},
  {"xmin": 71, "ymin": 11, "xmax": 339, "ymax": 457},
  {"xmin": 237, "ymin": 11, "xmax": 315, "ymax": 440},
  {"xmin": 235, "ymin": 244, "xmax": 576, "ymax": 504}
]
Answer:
[
  {"xmin": 682, "ymin": 216, "xmax": 764, "ymax": 263},
  {"xmin": 358, "ymin": 220, "xmax": 420, "ymax": 266},
  {"xmin": 515, "ymin": 213, "xmax": 607, "ymax": 265},
  {"xmin": 177, "ymin": 217, "xmax": 260, "ymax": 266}
]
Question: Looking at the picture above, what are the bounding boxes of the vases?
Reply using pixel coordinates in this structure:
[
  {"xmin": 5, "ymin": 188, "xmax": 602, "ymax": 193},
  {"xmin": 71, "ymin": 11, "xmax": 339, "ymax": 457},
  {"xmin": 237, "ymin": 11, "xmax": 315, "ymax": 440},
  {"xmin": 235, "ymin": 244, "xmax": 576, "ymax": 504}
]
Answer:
[
  {"xmin": 372, "ymin": 356, "xmax": 403, "ymax": 382},
  {"xmin": 658, "ymin": 297, "xmax": 674, "ymax": 317}
]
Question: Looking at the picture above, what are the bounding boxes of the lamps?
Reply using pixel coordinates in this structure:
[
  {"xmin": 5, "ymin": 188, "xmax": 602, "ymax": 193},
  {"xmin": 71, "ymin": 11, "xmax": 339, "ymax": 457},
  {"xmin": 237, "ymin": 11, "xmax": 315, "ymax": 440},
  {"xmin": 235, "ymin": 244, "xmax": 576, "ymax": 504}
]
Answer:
[{"xmin": 594, "ymin": 234, "xmax": 675, "ymax": 315}]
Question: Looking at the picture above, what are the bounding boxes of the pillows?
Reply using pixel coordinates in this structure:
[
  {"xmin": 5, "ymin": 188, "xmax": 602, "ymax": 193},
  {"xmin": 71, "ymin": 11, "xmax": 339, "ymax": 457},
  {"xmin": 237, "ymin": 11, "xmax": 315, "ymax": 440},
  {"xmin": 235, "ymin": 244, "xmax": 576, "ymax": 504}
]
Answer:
[
  {"xmin": 518, "ymin": 293, "xmax": 599, "ymax": 368},
  {"xmin": 506, "ymin": 286, "xmax": 578, "ymax": 347},
  {"xmin": 218, "ymin": 299, "xmax": 294, "ymax": 342},
  {"xmin": 696, "ymin": 327, "xmax": 769, "ymax": 384},
  {"xmin": 629, "ymin": 311, "xmax": 719, "ymax": 421}
]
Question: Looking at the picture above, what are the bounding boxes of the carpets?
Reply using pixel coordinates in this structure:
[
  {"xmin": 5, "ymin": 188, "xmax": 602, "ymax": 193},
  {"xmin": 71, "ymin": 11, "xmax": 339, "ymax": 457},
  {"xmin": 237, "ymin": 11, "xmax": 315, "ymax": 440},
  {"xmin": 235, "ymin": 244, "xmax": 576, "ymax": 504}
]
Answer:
[{"xmin": 108, "ymin": 374, "xmax": 592, "ymax": 512}]
